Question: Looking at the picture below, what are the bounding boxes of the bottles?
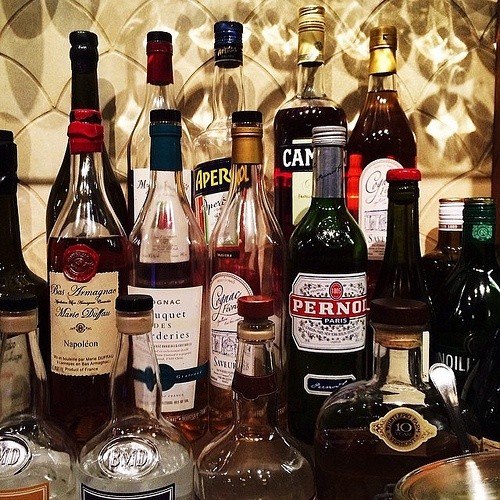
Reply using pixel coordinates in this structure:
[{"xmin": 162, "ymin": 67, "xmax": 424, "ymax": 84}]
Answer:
[{"xmin": 0, "ymin": 2, "xmax": 497, "ymax": 500}]
